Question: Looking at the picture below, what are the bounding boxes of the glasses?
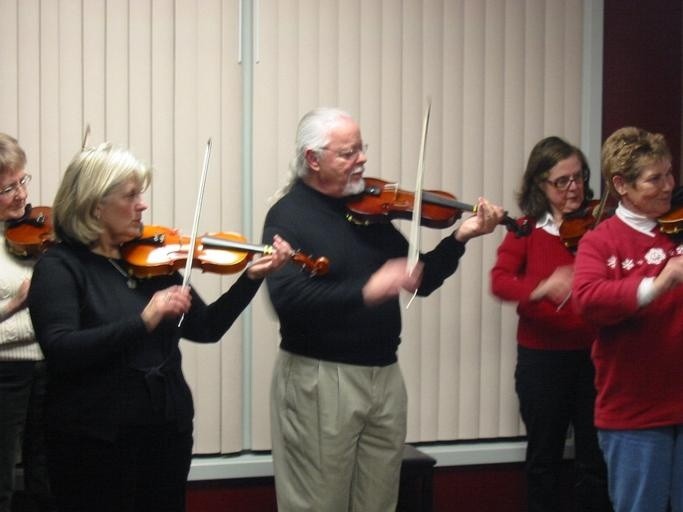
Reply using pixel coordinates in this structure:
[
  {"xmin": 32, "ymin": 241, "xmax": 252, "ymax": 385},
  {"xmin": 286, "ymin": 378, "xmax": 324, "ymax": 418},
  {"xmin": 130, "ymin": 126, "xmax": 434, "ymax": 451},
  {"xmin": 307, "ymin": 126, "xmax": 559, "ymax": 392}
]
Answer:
[
  {"xmin": 1, "ymin": 175, "xmax": 32, "ymax": 197},
  {"xmin": 322, "ymin": 144, "xmax": 369, "ymax": 159},
  {"xmin": 542, "ymin": 168, "xmax": 589, "ymax": 189}
]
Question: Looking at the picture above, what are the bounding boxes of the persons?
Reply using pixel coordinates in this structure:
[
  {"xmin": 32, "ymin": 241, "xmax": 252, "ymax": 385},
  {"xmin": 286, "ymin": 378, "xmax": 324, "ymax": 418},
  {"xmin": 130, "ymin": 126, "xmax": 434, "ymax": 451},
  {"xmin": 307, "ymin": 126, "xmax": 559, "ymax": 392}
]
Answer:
[
  {"xmin": 27, "ymin": 145, "xmax": 295, "ymax": 511},
  {"xmin": 0, "ymin": 132, "xmax": 45, "ymax": 511},
  {"xmin": 262, "ymin": 107, "xmax": 506, "ymax": 511},
  {"xmin": 571, "ymin": 126, "xmax": 683, "ymax": 512},
  {"xmin": 489, "ymin": 135, "xmax": 610, "ymax": 512}
]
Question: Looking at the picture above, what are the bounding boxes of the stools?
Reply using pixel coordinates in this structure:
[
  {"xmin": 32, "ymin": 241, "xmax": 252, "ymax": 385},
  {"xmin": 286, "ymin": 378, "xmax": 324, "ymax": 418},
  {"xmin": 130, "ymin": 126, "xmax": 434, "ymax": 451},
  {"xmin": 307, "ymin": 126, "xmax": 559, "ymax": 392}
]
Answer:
[{"xmin": 393, "ymin": 442, "xmax": 436, "ymax": 511}]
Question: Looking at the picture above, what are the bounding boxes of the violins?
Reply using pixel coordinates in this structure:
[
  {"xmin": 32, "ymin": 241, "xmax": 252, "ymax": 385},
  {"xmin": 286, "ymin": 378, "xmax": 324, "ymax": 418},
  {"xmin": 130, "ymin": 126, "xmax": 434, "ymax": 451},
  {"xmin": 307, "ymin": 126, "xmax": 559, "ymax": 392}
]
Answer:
[
  {"xmin": 657, "ymin": 196, "xmax": 683, "ymax": 235},
  {"xmin": 558, "ymin": 198, "xmax": 619, "ymax": 247},
  {"xmin": 5, "ymin": 203, "xmax": 56, "ymax": 259},
  {"xmin": 119, "ymin": 225, "xmax": 328, "ymax": 277},
  {"xmin": 343, "ymin": 176, "xmax": 531, "ymax": 239}
]
